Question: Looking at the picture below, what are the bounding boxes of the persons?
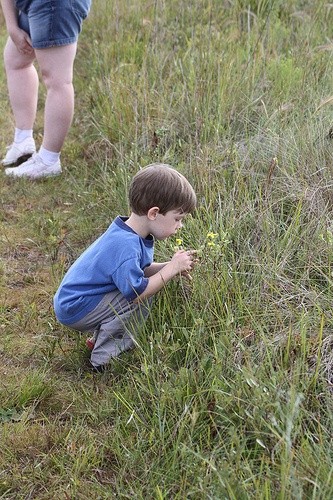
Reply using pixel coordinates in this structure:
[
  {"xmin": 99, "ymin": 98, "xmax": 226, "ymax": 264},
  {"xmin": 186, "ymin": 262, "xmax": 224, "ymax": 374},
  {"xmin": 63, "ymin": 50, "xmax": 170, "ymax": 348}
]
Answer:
[
  {"xmin": 54, "ymin": 162, "xmax": 198, "ymax": 374},
  {"xmin": 0, "ymin": 0, "xmax": 93, "ymax": 178}
]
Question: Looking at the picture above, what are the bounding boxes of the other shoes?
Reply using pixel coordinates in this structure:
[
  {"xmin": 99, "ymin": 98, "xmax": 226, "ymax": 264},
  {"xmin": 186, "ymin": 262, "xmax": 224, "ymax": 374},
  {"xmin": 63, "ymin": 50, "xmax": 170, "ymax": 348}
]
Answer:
[
  {"xmin": 87, "ymin": 359, "xmax": 111, "ymax": 374},
  {"xmin": 85, "ymin": 338, "xmax": 93, "ymax": 349}
]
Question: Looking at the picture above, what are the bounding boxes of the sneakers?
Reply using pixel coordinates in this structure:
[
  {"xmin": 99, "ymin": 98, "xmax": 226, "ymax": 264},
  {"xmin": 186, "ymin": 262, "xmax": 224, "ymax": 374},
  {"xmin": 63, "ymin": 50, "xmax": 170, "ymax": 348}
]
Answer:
[
  {"xmin": 0, "ymin": 137, "xmax": 36, "ymax": 165},
  {"xmin": 5, "ymin": 154, "xmax": 62, "ymax": 180}
]
